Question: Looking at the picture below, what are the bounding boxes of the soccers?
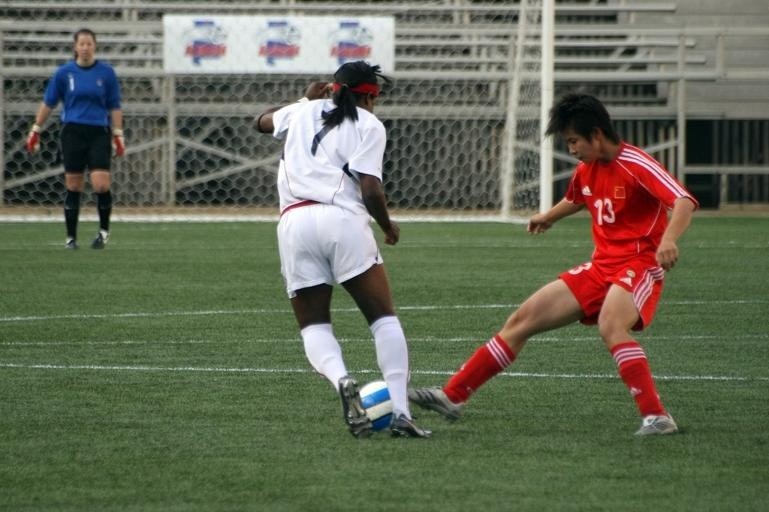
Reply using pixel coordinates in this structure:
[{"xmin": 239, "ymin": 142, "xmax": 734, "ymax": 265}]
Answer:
[{"xmin": 359, "ymin": 381, "xmax": 392, "ymax": 431}]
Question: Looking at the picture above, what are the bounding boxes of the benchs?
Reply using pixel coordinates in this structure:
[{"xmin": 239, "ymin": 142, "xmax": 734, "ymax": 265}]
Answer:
[{"xmin": 1, "ymin": 1, "xmax": 768, "ymax": 100}]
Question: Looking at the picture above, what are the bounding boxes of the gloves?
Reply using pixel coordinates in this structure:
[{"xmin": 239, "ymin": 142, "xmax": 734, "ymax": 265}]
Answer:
[
  {"xmin": 26, "ymin": 124, "xmax": 42, "ymax": 153},
  {"xmin": 112, "ymin": 128, "xmax": 124, "ymax": 159}
]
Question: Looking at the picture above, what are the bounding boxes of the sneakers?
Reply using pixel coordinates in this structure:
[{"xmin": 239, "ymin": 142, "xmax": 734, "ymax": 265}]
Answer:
[
  {"xmin": 408, "ymin": 385, "xmax": 465, "ymax": 422},
  {"xmin": 92, "ymin": 227, "xmax": 110, "ymax": 249},
  {"xmin": 388, "ymin": 410, "xmax": 434, "ymax": 439},
  {"xmin": 633, "ymin": 412, "xmax": 679, "ymax": 437},
  {"xmin": 66, "ymin": 237, "xmax": 79, "ymax": 249},
  {"xmin": 339, "ymin": 373, "xmax": 375, "ymax": 439}
]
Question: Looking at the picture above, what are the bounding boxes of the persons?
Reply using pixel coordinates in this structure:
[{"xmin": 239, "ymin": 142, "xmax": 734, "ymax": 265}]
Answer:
[
  {"xmin": 408, "ymin": 93, "xmax": 700, "ymax": 437},
  {"xmin": 252, "ymin": 60, "xmax": 434, "ymax": 437},
  {"xmin": 26, "ymin": 30, "xmax": 127, "ymax": 251}
]
